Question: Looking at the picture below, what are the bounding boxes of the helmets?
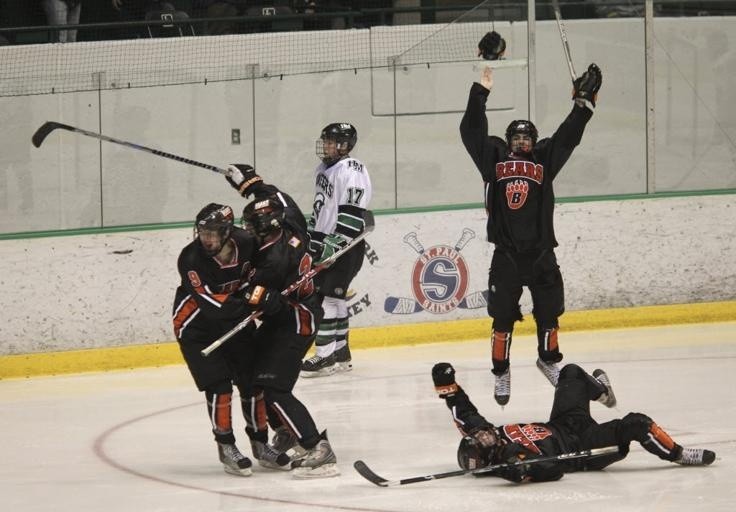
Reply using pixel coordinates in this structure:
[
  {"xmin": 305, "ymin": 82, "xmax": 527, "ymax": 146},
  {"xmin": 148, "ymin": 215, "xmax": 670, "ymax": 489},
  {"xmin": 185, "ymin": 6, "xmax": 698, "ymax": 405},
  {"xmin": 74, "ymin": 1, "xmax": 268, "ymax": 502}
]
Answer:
[
  {"xmin": 314, "ymin": 122, "xmax": 358, "ymax": 166},
  {"xmin": 505, "ymin": 119, "xmax": 538, "ymax": 156},
  {"xmin": 457, "ymin": 424, "xmax": 498, "ymax": 471},
  {"xmin": 192, "ymin": 202, "xmax": 234, "ymax": 254},
  {"xmin": 240, "ymin": 197, "xmax": 287, "ymax": 240}
]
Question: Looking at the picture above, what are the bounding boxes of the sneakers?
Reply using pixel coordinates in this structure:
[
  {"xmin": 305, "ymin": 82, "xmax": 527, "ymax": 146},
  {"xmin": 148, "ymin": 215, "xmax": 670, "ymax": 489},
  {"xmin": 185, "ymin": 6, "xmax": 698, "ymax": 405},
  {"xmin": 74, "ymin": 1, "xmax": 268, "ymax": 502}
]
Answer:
[
  {"xmin": 334, "ymin": 343, "xmax": 352, "ymax": 364},
  {"xmin": 212, "ymin": 430, "xmax": 252, "ymax": 470},
  {"xmin": 592, "ymin": 369, "xmax": 617, "ymax": 409},
  {"xmin": 300, "ymin": 352, "xmax": 337, "ymax": 371},
  {"xmin": 291, "ymin": 428, "xmax": 336, "ymax": 468},
  {"xmin": 277, "ymin": 434, "xmax": 312, "ymax": 466},
  {"xmin": 534, "ymin": 351, "xmax": 565, "ymax": 387},
  {"xmin": 494, "ymin": 368, "xmax": 512, "ymax": 406},
  {"xmin": 673, "ymin": 447, "xmax": 718, "ymax": 467},
  {"xmin": 245, "ymin": 426, "xmax": 291, "ymax": 467}
]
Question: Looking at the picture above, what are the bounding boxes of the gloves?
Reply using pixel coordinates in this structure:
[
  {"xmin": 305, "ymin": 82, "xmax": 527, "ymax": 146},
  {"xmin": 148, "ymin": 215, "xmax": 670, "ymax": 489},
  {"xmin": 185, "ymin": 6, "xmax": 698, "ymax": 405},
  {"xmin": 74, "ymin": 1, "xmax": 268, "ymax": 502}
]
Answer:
[
  {"xmin": 477, "ymin": 31, "xmax": 506, "ymax": 60},
  {"xmin": 492, "ymin": 464, "xmax": 526, "ymax": 483},
  {"xmin": 236, "ymin": 264, "xmax": 283, "ymax": 305},
  {"xmin": 306, "ymin": 230, "xmax": 338, "ymax": 273},
  {"xmin": 432, "ymin": 362, "xmax": 459, "ymax": 399},
  {"xmin": 572, "ymin": 62, "xmax": 604, "ymax": 109},
  {"xmin": 226, "ymin": 163, "xmax": 264, "ymax": 201},
  {"xmin": 241, "ymin": 284, "xmax": 288, "ymax": 319}
]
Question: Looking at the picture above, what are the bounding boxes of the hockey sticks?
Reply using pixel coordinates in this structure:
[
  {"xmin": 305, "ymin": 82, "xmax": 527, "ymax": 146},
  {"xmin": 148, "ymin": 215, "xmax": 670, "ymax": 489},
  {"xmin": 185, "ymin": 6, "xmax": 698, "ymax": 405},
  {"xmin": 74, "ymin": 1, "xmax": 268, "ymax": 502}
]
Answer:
[
  {"xmin": 355, "ymin": 446, "xmax": 620, "ymax": 488},
  {"xmin": 32, "ymin": 121, "xmax": 229, "ymax": 174},
  {"xmin": 550, "ymin": 0, "xmax": 583, "ymax": 104},
  {"xmin": 201, "ymin": 209, "xmax": 375, "ymax": 355}
]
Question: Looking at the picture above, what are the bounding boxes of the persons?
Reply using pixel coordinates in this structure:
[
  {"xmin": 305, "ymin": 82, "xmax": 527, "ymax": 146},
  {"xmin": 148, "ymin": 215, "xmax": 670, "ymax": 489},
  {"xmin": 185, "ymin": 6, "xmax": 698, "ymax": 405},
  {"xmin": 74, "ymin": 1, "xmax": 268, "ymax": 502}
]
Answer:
[
  {"xmin": 457, "ymin": 62, "xmax": 603, "ymax": 406},
  {"xmin": 431, "ymin": 360, "xmax": 713, "ymax": 481},
  {"xmin": 175, "ymin": 204, "xmax": 290, "ymax": 473},
  {"xmin": 224, "ymin": 162, "xmax": 338, "ymax": 477},
  {"xmin": 43, "ymin": 0, "xmax": 84, "ymax": 42},
  {"xmin": 297, "ymin": 124, "xmax": 369, "ymax": 375}
]
